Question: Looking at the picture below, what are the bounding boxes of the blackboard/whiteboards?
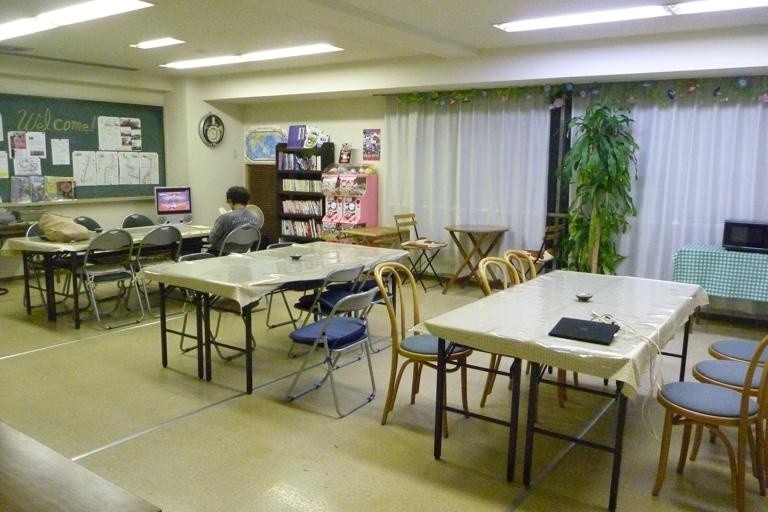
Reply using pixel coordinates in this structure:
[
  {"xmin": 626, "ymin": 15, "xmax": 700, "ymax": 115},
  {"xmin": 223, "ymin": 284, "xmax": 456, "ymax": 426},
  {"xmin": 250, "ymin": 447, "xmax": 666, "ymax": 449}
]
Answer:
[{"xmin": 0, "ymin": 92, "xmax": 166, "ymax": 208}]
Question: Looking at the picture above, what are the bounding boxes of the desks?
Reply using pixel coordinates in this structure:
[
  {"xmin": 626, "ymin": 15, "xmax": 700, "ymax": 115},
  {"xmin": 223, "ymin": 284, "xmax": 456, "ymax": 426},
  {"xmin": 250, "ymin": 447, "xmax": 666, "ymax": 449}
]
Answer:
[{"xmin": 1, "ymin": 223, "xmax": 212, "ymax": 329}]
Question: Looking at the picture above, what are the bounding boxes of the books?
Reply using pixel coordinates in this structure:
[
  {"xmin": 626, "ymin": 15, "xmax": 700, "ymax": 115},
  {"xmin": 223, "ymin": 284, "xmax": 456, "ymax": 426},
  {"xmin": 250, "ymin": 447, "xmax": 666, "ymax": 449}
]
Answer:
[
  {"xmin": 316, "ymin": 134, "xmax": 329, "ymax": 149},
  {"xmin": 287, "ymin": 125, "xmax": 310, "ymax": 149},
  {"xmin": 278, "ymin": 151, "xmax": 324, "ymax": 244}
]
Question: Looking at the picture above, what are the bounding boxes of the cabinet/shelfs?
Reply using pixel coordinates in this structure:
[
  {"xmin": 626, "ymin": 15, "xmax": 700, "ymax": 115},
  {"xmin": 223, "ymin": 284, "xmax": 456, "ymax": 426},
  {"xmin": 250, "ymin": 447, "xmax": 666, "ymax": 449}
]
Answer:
[
  {"xmin": 322, "ymin": 161, "xmax": 378, "ymax": 247},
  {"xmin": 276, "ymin": 143, "xmax": 334, "ymax": 241}
]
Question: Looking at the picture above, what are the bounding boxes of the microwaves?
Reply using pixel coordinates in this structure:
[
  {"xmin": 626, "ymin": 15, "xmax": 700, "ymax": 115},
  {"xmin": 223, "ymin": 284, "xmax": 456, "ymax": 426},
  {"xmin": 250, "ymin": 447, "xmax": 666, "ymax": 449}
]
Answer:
[{"xmin": 721, "ymin": 220, "xmax": 768, "ymax": 255}]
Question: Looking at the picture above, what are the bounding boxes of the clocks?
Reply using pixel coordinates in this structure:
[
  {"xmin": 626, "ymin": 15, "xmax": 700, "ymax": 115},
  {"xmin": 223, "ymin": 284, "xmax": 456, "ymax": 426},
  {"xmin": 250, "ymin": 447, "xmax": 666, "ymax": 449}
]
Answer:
[{"xmin": 199, "ymin": 112, "xmax": 226, "ymax": 147}]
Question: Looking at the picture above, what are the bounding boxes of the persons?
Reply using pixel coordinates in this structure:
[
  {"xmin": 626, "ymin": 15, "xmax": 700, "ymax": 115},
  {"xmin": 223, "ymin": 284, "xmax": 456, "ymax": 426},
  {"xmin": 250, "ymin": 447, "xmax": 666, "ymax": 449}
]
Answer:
[
  {"xmin": 206, "ymin": 185, "xmax": 259, "ymax": 256},
  {"xmin": 10, "ymin": 135, "xmax": 26, "ymax": 158}
]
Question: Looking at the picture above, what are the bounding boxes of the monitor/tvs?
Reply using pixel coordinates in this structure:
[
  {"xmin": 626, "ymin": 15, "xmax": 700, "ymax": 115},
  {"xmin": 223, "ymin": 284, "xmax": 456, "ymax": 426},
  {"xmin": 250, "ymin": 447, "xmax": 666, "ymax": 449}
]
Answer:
[{"xmin": 154, "ymin": 186, "xmax": 192, "ymax": 224}]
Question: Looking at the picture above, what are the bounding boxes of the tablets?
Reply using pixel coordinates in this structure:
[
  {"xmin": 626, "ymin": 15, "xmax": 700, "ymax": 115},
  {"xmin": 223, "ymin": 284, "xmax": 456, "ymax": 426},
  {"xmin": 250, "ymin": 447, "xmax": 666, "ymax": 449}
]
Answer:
[{"xmin": 548, "ymin": 317, "xmax": 620, "ymax": 346}]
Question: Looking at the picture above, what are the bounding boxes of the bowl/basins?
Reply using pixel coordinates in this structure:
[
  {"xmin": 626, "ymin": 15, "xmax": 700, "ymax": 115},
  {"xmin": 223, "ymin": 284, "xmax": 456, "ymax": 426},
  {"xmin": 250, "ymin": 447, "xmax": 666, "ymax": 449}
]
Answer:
[
  {"xmin": 576, "ymin": 292, "xmax": 592, "ymax": 303},
  {"xmin": 290, "ymin": 254, "xmax": 302, "ymax": 260}
]
